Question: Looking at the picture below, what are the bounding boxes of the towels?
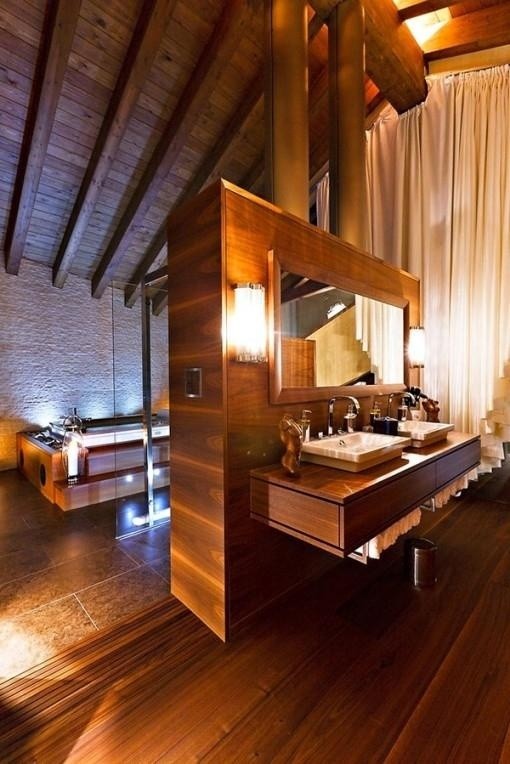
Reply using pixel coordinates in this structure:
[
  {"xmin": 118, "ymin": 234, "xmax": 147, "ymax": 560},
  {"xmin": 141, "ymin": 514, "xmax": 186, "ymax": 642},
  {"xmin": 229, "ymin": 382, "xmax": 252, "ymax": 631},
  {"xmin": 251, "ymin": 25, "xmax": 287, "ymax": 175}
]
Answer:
[{"xmin": 353, "ymin": 473, "xmax": 471, "ymax": 558}]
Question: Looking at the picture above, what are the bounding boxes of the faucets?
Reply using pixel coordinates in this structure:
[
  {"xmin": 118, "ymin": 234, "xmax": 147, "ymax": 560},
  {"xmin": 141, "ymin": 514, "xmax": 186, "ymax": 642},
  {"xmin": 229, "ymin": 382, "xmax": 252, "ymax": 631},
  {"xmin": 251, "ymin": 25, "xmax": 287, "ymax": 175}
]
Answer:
[
  {"xmin": 387, "ymin": 391, "xmax": 416, "ymax": 417},
  {"xmin": 327, "ymin": 394, "xmax": 361, "ymax": 433}
]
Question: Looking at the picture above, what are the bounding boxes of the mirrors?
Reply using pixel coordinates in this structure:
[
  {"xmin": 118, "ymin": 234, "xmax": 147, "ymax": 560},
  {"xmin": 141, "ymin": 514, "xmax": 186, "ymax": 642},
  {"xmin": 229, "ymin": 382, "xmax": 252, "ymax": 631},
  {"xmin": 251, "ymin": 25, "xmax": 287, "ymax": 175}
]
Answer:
[{"xmin": 266, "ymin": 249, "xmax": 410, "ymax": 405}]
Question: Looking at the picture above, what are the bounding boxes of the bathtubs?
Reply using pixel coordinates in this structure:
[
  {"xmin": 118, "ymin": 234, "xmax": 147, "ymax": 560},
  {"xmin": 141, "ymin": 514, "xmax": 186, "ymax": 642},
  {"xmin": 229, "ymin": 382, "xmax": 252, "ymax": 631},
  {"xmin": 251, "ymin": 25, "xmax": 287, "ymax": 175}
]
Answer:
[{"xmin": 49, "ymin": 419, "xmax": 171, "ymax": 447}]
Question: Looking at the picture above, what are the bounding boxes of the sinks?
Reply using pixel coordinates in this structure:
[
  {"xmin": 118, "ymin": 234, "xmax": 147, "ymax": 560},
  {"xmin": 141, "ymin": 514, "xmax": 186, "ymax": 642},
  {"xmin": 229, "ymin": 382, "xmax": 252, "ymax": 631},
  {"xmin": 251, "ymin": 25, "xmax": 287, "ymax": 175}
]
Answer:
[
  {"xmin": 395, "ymin": 420, "xmax": 456, "ymax": 448},
  {"xmin": 300, "ymin": 430, "xmax": 413, "ymax": 473}
]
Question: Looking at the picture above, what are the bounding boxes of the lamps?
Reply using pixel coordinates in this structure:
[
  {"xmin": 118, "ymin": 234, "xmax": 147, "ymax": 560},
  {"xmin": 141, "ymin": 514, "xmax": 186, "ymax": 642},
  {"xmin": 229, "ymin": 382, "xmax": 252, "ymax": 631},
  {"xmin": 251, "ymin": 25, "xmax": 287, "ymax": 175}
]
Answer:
[
  {"xmin": 230, "ymin": 279, "xmax": 274, "ymax": 368},
  {"xmin": 408, "ymin": 325, "xmax": 429, "ymax": 371}
]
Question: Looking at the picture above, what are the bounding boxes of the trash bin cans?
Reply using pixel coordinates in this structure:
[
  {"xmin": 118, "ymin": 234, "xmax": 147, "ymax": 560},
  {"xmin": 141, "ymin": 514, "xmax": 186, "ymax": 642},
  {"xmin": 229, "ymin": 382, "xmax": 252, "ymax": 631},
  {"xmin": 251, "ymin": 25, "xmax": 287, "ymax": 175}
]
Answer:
[{"xmin": 404, "ymin": 537, "xmax": 438, "ymax": 590}]
